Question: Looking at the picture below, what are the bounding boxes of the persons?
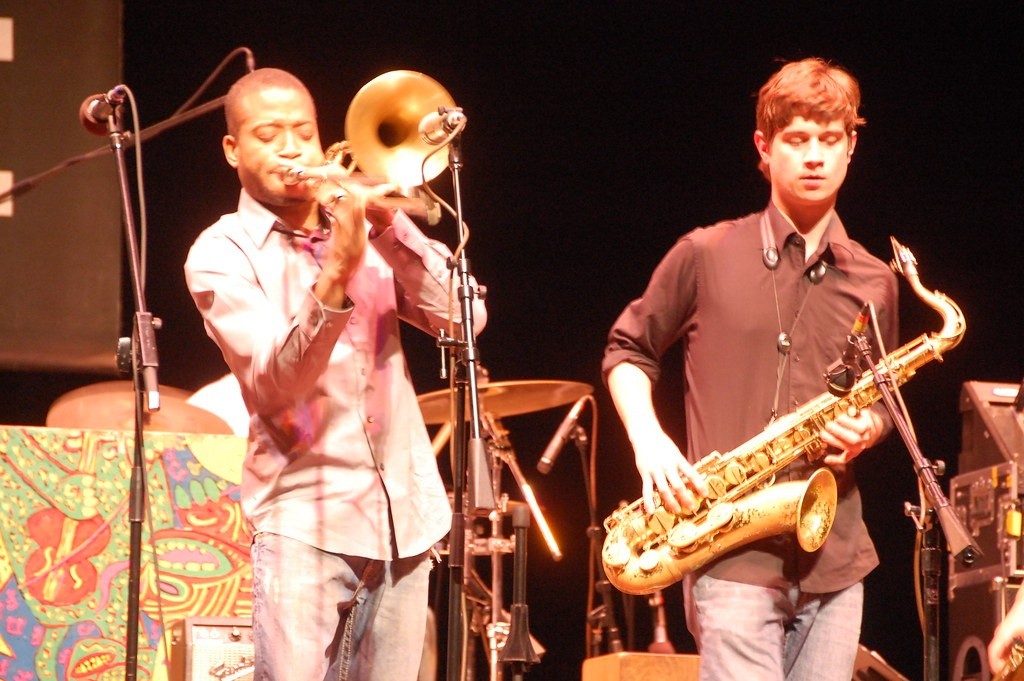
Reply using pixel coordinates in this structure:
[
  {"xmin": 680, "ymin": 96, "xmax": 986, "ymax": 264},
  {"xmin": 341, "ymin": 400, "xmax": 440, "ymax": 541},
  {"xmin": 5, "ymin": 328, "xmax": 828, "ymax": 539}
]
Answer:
[
  {"xmin": 987, "ymin": 579, "xmax": 1024, "ymax": 675},
  {"xmin": 184, "ymin": 68, "xmax": 487, "ymax": 681},
  {"xmin": 600, "ymin": 59, "xmax": 899, "ymax": 681}
]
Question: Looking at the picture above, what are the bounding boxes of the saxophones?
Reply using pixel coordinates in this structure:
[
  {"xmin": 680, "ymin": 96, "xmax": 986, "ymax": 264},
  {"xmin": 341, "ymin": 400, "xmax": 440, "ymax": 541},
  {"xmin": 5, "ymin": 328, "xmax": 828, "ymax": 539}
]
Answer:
[{"xmin": 599, "ymin": 231, "xmax": 972, "ymax": 598}]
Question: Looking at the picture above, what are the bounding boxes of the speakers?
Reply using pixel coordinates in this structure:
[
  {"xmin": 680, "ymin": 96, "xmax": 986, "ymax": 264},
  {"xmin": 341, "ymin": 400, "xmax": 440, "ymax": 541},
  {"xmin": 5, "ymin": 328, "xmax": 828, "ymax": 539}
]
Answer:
[{"xmin": 947, "ymin": 376, "xmax": 1024, "ymax": 681}]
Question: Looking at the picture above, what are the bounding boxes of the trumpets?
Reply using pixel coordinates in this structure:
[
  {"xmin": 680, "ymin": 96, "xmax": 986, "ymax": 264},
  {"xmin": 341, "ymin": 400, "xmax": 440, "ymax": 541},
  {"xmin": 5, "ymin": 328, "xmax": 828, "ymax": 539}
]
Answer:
[{"xmin": 273, "ymin": 68, "xmax": 459, "ymax": 224}]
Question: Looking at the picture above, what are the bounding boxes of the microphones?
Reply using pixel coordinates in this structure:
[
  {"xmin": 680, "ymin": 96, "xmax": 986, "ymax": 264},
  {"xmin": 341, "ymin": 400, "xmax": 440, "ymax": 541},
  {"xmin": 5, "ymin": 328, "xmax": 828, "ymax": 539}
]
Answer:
[
  {"xmin": 536, "ymin": 393, "xmax": 590, "ymax": 476},
  {"xmin": 821, "ymin": 301, "xmax": 874, "ymax": 398},
  {"xmin": 77, "ymin": 84, "xmax": 128, "ymax": 137},
  {"xmin": 418, "ymin": 107, "xmax": 466, "ymax": 147}
]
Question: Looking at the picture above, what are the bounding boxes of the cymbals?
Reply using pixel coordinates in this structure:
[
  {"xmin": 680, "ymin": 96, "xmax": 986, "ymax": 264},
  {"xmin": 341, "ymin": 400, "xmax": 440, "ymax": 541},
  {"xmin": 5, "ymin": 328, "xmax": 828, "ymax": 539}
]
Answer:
[
  {"xmin": 417, "ymin": 374, "xmax": 593, "ymax": 427},
  {"xmin": 45, "ymin": 381, "xmax": 231, "ymax": 433}
]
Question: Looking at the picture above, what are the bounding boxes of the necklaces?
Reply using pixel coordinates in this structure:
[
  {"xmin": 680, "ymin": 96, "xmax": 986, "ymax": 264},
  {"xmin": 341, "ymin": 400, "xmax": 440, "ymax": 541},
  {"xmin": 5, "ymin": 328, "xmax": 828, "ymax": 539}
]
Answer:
[
  {"xmin": 755, "ymin": 211, "xmax": 828, "ymax": 489},
  {"xmin": 273, "ymin": 227, "xmax": 330, "ymax": 238}
]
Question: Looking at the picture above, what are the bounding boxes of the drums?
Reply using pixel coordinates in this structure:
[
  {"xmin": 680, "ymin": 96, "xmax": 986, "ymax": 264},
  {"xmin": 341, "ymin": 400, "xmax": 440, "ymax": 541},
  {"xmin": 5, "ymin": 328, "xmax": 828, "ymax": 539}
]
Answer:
[{"xmin": 432, "ymin": 498, "xmax": 530, "ymax": 557}]
